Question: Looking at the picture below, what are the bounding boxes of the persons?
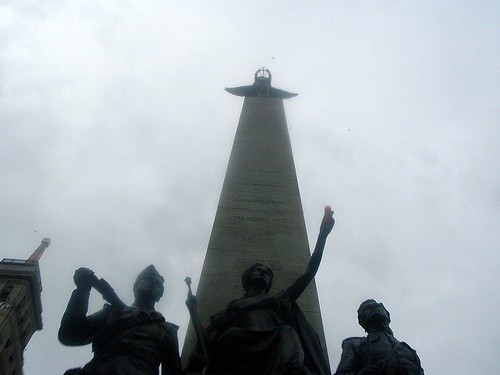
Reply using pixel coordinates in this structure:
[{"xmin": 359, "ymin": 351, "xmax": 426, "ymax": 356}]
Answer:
[
  {"xmin": 56, "ymin": 264, "xmax": 184, "ymax": 375},
  {"xmin": 333, "ymin": 299, "xmax": 425, "ymax": 375},
  {"xmin": 183, "ymin": 207, "xmax": 334, "ymax": 375}
]
